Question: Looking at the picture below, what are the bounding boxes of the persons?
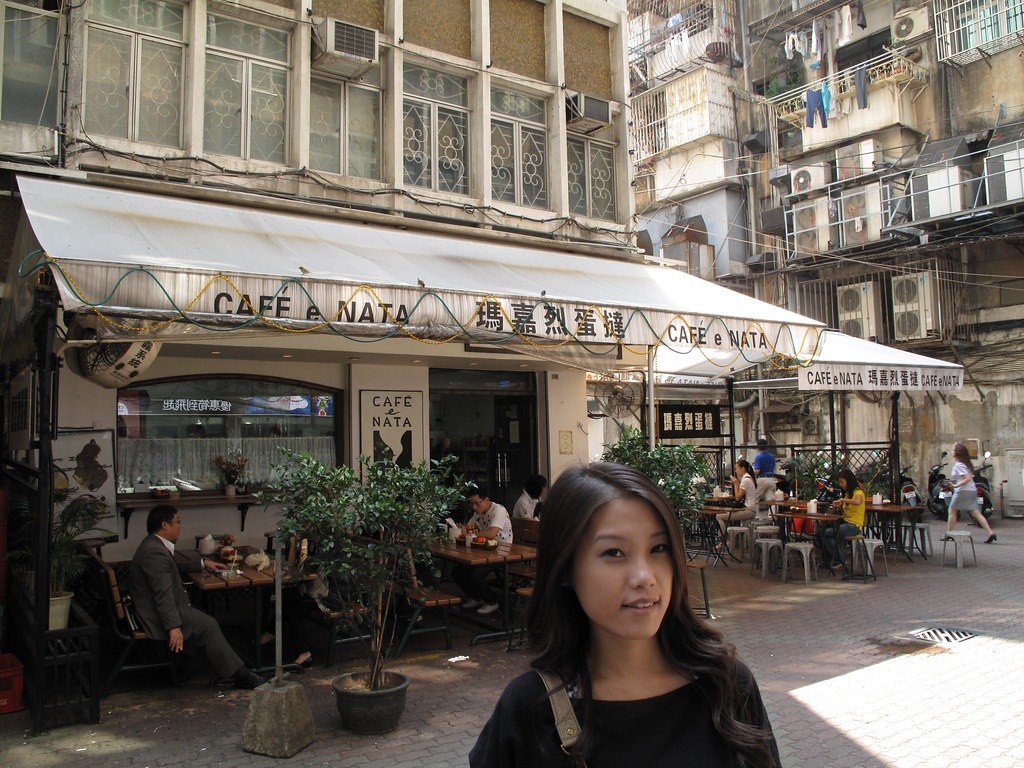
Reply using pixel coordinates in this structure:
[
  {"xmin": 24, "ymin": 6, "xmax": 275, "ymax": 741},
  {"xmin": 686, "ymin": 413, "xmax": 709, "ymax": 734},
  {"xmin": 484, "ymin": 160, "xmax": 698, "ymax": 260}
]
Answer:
[
  {"xmin": 715, "ymin": 439, "xmax": 778, "ymax": 550},
  {"xmin": 468, "ymin": 462, "xmax": 783, "ymax": 768},
  {"xmin": 128, "ymin": 504, "xmax": 267, "ymax": 690},
  {"xmin": 816, "ymin": 469, "xmax": 866, "ymax": 568},
  {"xmin": 513, "ymin": 474, "xmax": 548, "ymax": 564},
  {"xmin": 451, "ymin": 487, "xmax": 514, "ymax": 614},
  {"xmin": 939, "ymin": 444, "xmax": 998, "ymax": 543},
  {"xmin": 252, "ymin": 535, "xmax": 351, "ymax": 672}
]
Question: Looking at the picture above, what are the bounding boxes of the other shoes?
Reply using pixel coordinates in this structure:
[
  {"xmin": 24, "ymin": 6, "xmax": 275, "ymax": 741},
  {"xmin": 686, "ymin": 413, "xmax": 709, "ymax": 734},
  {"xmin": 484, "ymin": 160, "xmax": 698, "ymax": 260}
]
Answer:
[
  {"xmin": 285, "ymin": 654, "xmax": 313, "ymax": 671},
  {"xmin": 250, "ymin": 631, "xmax": 275, "ymax": 647},
  {"xmin": 477, "ymin": 603, "xmax": 498, "ymax": 614},
  {"xmin": 462, "ymin": 599, "xmax": 484, "ymax": 608},
  {"xmin": 715, "ymin": 539, "xmax": 730, "ymax": 549},
  {"xmin": 235, "ymin": 669, "xmax": 268, "ymax": 689},
  {"xmin": 831, "ymin": 560, "xmax": 848, "ymax": 570}
]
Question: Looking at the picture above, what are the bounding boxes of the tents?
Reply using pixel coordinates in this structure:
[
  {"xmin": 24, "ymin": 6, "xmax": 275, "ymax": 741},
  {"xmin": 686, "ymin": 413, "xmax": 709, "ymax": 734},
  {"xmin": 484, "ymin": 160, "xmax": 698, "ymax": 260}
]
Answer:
[{"xmin": 586, "ymin": 329, "xmax": 965, "ymax": 550}]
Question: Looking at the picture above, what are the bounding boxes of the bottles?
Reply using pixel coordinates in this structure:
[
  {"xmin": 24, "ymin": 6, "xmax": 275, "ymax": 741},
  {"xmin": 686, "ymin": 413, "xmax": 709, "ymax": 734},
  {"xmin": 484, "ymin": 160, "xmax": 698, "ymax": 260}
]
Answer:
[
  {"xmin": 436, "ymin": 413, "xmax": 489, "ymax": 480},
  {"xmin": 439, "ymin": 509, "xmax": 479, "ymax": 550},
  {"xmin": 220, "ymin": 541, "xmax": 234, "ymax": 558},
  {"xmin": 730, "ymin": 475, "xmax": 740, "ymax": 487}
]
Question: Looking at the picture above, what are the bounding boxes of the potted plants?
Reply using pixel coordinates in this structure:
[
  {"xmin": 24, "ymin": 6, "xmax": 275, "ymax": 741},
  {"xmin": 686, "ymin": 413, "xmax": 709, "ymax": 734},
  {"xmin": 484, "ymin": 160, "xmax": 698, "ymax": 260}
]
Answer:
[{"xmin": 251, "ymin": 442, "xmax": 487, "ymax": 740}]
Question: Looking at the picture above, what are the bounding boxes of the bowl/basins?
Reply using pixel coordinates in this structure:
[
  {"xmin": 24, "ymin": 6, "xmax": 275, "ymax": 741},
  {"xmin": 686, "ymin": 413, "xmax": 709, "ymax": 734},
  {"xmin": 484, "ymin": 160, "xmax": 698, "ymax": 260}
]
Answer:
[
  {"xmin": 455, "ymin": 537, "xmax": 465, "ymax": 546},
  {"xmin": 471, "ymin": 542, "xmax": 485, "ymax": 549}
]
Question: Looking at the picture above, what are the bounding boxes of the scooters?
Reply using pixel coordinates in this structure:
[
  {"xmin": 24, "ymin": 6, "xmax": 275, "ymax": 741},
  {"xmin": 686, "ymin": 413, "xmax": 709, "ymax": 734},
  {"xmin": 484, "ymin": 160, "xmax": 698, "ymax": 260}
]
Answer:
[
  {"xmin": 965, "ymin": 450, "xmax": 993, "ymax": 526},
  {"xmin": 927, "ymin": 451, "xmax": 961, "ymax": 522},
  {"xmin": 882, "ymin": 466, "xmax": 924, "ymax": 523}
]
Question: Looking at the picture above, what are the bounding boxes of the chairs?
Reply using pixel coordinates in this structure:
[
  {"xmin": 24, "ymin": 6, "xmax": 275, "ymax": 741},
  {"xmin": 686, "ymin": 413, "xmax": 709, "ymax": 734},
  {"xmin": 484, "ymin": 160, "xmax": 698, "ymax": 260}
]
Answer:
[{"xmin": 685, "ymin": 524, "xmax": 719, "ymax": 621}]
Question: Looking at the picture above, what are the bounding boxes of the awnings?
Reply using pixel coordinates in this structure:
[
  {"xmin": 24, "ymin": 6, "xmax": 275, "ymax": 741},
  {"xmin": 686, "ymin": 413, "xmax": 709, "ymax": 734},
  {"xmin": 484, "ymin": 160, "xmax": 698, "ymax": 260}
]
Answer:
[{"xmin": 11, "ymin": 175, "xmax": 829, "ymax": 356}]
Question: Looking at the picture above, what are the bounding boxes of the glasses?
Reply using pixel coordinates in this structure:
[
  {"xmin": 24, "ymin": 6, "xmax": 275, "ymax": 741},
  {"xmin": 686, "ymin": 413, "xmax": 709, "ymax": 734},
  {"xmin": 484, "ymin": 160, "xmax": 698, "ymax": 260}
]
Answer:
[
  {"xmin": 468, "ymin": 496, "xmax": 486, "ymax": 506},
  {"xmin": 170, "ymin": 519, "xmax": 180, "ymax": 524}
]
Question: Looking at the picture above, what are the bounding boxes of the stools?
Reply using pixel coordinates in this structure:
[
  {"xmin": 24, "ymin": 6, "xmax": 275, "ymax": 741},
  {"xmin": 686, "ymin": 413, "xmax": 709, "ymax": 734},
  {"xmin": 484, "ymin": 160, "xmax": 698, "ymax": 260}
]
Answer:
[
  {"xmin": 939, "ymin": 527, "xmax": 979, "ymax": 570},
  {"xmin": 840, "ymin": 534, "xmax": 877, "ymax": 584},
  {"xmin": 721, "ymin": 524, "xmax": 751, "ymax": 560},
  {"xmin": 851, "ymin": 538, "xmax": 888, "ymax": 576},
  {"xmin": 750, "ymin": 538, "xmax": 786, "ymax": 580},
  {"xmin": 739, "ymin": 517, "xmax": 760, "ymax": 552},
  {"xmin": 752, "ymin": 523, "xmax": 780, "ymax": 570},
  {"xmin": 780, "ymin": 541, "xmax": 818, "ymax": 587},
  {"xmin": 900, "ymin": 519, "xmax": 935, "ymax": 557}
]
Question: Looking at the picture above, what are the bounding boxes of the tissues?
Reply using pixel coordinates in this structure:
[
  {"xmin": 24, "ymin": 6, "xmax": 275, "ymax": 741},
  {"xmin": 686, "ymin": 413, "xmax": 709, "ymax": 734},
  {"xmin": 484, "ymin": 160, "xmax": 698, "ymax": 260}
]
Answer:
[
  {"xmin": 806, "ymin": 499, "xmax": 818, "ymax": 514},
  {"xmin": 774, "ymin": 489, "xmax": 785, "ymax": 501},
  {"xmin": 199, "ymin": 534, "xmax": 215, "ymax": 553},
  {"xmin": 713, "ymin": 485, "xmax": 721, "ymax": 495},
  {"xmin": 446, "ymin": 518, "xmax": 463, "ymax": 541},
  {"xmin": 872, "ymin": 492, "xmax": 883, "ymax": 505}
]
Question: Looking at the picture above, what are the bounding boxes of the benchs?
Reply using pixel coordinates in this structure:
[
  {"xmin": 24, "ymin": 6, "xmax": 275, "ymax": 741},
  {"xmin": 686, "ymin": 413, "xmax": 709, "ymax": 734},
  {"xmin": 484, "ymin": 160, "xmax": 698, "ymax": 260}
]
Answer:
[
  {"xmin": 263, "ymin": 522, "xmax": 391, "ymax": 668},
  {"xmin": 439, "ymin": 512, "xmax": 563, "ymax": 614},
  {"xmin": 79, "ymin": 536, "xmax": 200, "ymax": 699},
  {"xmin": 347, "ymin": 523, "xmax": 462, "ymax": 654}
]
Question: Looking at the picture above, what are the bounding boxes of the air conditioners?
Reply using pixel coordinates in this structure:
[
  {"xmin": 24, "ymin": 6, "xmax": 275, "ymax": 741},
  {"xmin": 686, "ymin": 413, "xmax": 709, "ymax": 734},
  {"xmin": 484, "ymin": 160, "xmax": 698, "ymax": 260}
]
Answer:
[
  {"xmin": 564, "ymin": 87, "xmax": 615, "ymax": 138},
  {"xmin": 835, "ymin": 137, "xmax": 885, "ymax": 179},
  {"xmin": 311, "ymin": 14, "xmax": 381, "ymax": 84},
  {"xmin": 892, "ymin": 269, "xmax": 939, "ymax": 342},
  {"xmin": 792, "ymin": 195, "xmax": 836, "ymax": 255},
  {"xmin": 790, "ymin": 161, "xmax": 833, "ymax": 191},
  {"xmin": 890, "ymin": 7, "xmax": 933, "ymax": 46},
  {"xmin": 841, "ymin": 179, "xmax": 900, "ymax": 250},
  {"xmin": 834, "ymin": 280, "xmax": 883, "ymax": 345}
]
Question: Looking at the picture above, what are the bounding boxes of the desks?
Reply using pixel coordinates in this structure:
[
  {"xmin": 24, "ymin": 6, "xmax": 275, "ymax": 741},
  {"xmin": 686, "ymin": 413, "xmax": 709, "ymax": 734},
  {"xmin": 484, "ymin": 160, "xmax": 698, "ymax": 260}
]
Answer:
[
  {"xmin": 865, "ymin": 501, "xmax": 929, "ymax": 563},
  {"xmin": 765, "ymin": 510, "xmax": 848, "ymax": 578},
  {"xmin": 173, "ymin": 544, "xmax": 313, "ymax": 687},
  {"xmin": 428, "ymin": 531, "xmax": 546, "ymax": 648},
  {"xmin": 683, "ymin": 500, "xmax": 750, "ymax": 568},
  {"xmin": 742, "ymin": 496, "xmax": 831, "ymax": 562}
]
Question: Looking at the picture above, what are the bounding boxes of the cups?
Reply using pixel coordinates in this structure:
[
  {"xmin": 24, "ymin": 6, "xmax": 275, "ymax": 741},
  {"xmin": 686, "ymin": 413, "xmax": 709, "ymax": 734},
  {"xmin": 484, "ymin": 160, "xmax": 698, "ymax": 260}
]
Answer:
[{"xmin": 222, "ymin": 555, "xmax": 243, "ymax": 581}]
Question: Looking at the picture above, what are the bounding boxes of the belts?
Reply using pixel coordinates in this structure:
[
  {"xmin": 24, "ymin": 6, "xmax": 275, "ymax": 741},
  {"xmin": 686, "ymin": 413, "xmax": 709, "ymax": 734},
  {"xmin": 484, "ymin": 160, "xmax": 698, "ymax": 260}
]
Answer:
[{"xmin": 756, "ymin": 475, "xmax": 773, "ymax": 477}]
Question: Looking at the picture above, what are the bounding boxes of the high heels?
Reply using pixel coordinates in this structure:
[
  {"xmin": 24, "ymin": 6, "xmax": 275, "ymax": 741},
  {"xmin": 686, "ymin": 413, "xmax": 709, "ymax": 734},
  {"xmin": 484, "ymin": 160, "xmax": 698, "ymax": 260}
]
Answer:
[
  {"xmin": 984, "ymin": 533, "xmax": 996, "ymax": 543},
  {"xmin": 940, "ymin": 535, "xmax": 954, "ymax": 541}
]
